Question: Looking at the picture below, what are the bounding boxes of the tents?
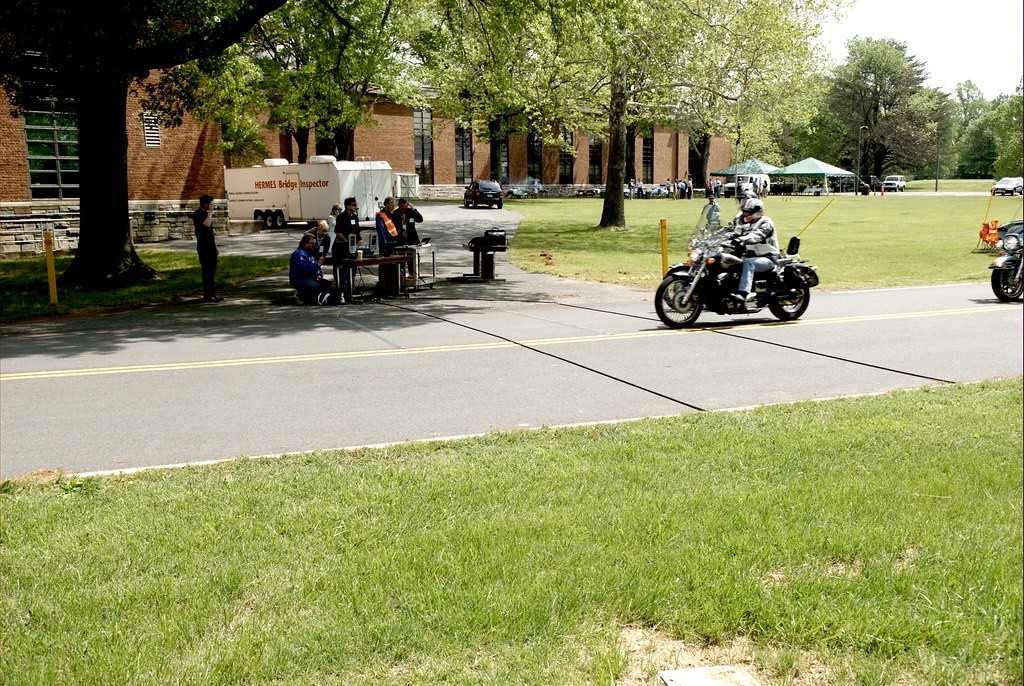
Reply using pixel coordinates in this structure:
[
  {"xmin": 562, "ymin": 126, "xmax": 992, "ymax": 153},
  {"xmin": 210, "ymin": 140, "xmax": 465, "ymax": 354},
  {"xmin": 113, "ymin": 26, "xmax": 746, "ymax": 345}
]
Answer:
[
  {"xmin": 774, "ymin": 158, "xmax": 856, "ymax": 195},
  {"xmin": 710, "ymin": 157, "xmax": 780, "ymax": 194}
]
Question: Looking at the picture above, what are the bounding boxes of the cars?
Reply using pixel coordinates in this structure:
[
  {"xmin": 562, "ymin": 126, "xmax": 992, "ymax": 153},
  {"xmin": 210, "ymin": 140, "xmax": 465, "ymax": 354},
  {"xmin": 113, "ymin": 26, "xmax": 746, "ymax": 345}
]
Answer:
[
  {"xmin": 834, "ymin": 178, "xmax": 856, "ymax": 193},
  {"xmin": 990, "ymin": 177, "xmax": 1024, "ymax": 196}
]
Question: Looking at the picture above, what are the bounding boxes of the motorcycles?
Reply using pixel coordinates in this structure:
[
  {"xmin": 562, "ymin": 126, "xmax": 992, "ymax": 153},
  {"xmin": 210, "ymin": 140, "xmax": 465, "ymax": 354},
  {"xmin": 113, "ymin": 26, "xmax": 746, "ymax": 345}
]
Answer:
[{"xmin": 653, "ymin": 202, "xmax": 820, "ymax": 329}]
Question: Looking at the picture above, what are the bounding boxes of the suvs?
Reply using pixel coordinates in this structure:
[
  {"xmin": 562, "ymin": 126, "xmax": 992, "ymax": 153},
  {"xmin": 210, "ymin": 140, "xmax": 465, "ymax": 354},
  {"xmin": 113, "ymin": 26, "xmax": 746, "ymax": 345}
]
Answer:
[{"xmin": 464, "ymin": 179, "xmax": 504, "ymax": 209}]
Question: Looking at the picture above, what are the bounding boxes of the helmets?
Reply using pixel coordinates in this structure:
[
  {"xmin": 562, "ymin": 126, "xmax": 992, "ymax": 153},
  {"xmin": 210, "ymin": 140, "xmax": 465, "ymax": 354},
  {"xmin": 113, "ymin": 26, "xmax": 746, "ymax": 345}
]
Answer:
[{"xmin": 740, "ymin": 200, "xmax": 765, "ymax": 223}]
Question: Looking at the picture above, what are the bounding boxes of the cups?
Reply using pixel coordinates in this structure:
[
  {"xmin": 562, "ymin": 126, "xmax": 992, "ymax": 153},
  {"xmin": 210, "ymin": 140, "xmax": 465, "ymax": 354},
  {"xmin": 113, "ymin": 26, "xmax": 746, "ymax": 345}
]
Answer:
[{"xmin": 357, "ymin": 250, "xmax": 363, "ymax": 261}]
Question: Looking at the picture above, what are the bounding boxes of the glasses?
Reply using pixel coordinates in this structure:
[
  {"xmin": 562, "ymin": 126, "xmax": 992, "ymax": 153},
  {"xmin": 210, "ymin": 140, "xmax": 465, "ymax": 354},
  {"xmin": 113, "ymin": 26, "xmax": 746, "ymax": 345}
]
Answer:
[{"xmin": 350, "ymin": 203, "xmax": 357, "ymax": 206}]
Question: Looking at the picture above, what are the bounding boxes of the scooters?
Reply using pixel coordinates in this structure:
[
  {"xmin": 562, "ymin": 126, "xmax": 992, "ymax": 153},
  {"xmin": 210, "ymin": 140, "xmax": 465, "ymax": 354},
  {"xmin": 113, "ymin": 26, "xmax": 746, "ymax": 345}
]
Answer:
[{"xmin": 987, "ymin": 218, "xmax": 1024, "ymax": 302}]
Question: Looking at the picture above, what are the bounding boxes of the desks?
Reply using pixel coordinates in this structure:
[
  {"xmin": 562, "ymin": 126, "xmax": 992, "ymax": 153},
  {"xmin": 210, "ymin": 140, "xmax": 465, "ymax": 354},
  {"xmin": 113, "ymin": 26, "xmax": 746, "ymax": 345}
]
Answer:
[{"xmin": 319, "ymin": 254, "xmax": 416, "ymax": 300}]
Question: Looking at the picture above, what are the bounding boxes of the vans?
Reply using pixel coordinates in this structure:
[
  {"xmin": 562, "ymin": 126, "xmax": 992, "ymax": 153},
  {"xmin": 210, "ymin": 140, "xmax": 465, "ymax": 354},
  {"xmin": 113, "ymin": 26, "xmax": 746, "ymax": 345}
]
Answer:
[
  {"xmin": 724, "ymin": 173, "xmax": 771, "ymax": 197},
  {"xmin": 861, "ymin": 175, "xmax": 883, "ymax": 192},
  {"xmin": 882, "ymin": 175, "xmax": 907, "ymax": 192}
]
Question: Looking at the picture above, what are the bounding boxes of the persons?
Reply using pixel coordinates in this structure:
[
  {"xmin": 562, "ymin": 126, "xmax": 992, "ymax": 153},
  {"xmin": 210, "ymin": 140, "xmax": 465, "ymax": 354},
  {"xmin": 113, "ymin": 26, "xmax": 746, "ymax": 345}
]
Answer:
[
  {"xmin": 705, "ymin": 177, "xmax": 721, "ymax": 198},
  {"xmin": 701, "ymin": 195, "xmax": 721, "ymax": 224},
  {"xmin": 753, "ymin": 178, "xmax": 758, "ymax": 194},
  {"xmin": 332, "ymin": 198, "xmax": 363, "ymax": 292},
  {"xmin": 629, "ymin": 178, "xmax": 645, "ymax": 200},
  {"xmin": 713, "ymin": 190, "xmax": 779, "ymax": 301},
  {"xmin": 328, "ymin": 203, "xmax": 342, "ymax": 254},
  {"xmin": 666, "ymin": 178, "xmax": 671, "ymax": 198},
  {"xmin": 304, "ymin": 220, "xmax": 330, "ymax": 257},
  {"xmin": 289, "ymin": 234, "xmax": 346, "ymax": 305},
  {"xmin": 761, "ymin": 180, "xmax": 767, "ymax": 198},
  {"xmin": 191, "ymin": 195, "xmax": 225, "ymax": 303},
  {"xmin": 674, "ymin": 178, "xmax": 692, "ymax": 200},
  {"xmin": 376, "ymin": 196, "xmax": 423, "ymax": 293},
  {"xmin": 737, "ymin": 177, "xmax": 743, "ymax": 194}
]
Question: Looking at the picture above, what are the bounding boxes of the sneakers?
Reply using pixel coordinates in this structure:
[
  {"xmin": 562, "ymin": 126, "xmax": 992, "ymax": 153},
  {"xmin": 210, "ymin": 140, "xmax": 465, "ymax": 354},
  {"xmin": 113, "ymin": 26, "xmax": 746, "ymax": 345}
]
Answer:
[
  {"xmin": 294, "ymin": 292, "xmax": 305, "ymax": 305},
  {"xmin": 204, "ymin": 295, "xmax": 224, "ymax": 303}
]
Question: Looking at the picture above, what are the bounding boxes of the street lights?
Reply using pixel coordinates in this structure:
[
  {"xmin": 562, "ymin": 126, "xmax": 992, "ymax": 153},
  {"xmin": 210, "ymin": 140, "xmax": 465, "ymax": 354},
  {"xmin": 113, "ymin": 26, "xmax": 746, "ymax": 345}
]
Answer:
[{"xmin": 856, "ymin": 126, "xmax": 868, "ymax": 197}]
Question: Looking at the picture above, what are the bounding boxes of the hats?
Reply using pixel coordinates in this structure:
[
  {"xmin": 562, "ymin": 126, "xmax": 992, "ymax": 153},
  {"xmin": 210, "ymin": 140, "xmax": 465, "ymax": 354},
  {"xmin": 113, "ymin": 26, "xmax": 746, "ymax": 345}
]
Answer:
[
  {"xmin": 709, "ymin": 195, "xmax": 714, "ymax": 199},
  {"xmin": 397, "ymin": 198, "xmax": 408, "ymax": 206}
]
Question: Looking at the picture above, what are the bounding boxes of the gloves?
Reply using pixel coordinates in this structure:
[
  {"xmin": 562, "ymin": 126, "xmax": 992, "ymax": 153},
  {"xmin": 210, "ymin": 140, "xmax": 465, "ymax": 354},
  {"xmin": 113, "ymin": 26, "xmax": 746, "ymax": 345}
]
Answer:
[{"xmin": 731, "ymin": 238, "xmax": 743, "ymax": 247}]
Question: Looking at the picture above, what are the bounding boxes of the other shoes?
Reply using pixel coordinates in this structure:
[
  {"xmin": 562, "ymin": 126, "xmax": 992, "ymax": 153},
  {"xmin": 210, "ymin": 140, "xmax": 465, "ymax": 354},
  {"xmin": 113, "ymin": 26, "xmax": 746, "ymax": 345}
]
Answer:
[
  {"xmin": 731, "ymin": 293, "xmax": 748, "ymax": 302},
  {"xmin": 409, "ymin": 274, "xmax": 414, "ymax": 279}
]
